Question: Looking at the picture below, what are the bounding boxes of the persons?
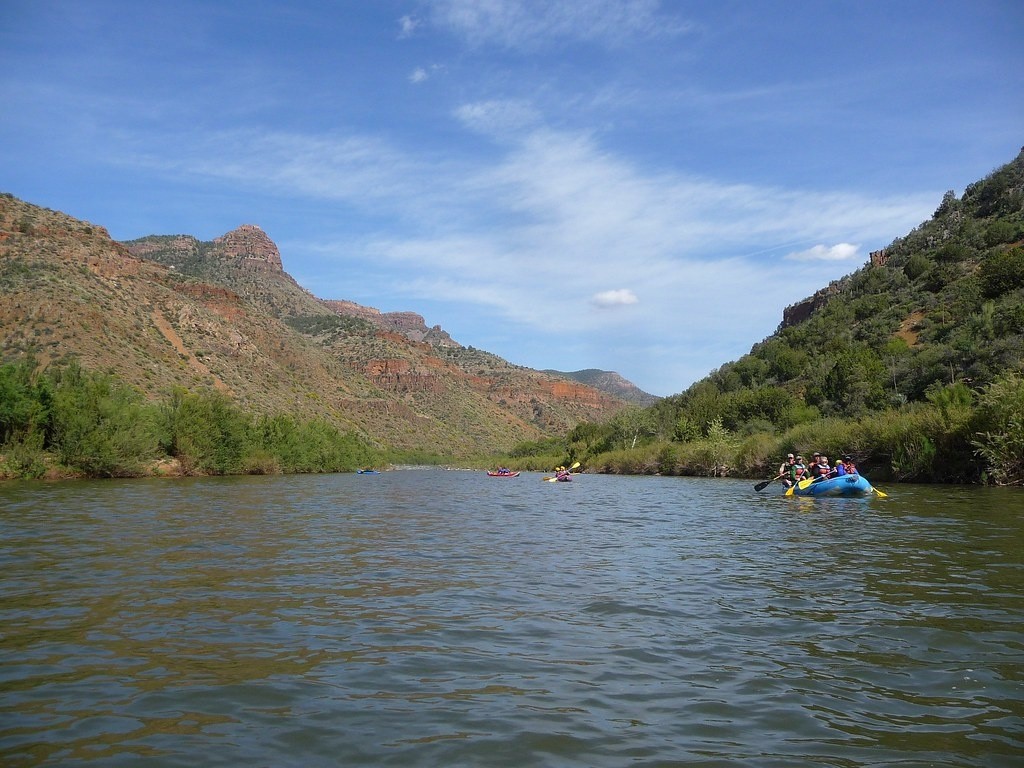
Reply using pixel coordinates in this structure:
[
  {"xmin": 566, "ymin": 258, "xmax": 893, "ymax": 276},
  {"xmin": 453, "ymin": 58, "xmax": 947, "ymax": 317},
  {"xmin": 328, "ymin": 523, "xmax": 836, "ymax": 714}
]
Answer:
[
  {"xmin": 779, "ymin": 452, "xmax": 858, "ymax": 488},
  {"xmin": 555, "ymin": 466, "xmax": 567, "ymax": 477},
  {"xmin": 498, "ymin": 466, "xmax": 510, "ymax": 474}
]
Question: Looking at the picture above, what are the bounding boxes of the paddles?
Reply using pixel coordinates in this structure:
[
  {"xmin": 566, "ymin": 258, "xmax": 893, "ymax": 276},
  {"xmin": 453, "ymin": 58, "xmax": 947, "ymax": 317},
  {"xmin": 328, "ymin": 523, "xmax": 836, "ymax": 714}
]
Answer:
[
  {"xmin": 548, "ymin": 461, "xmax": 581, "ymax": 483},
  {"xmin": 872, "ymin": 486, "xmax": 888, "ymax": 498},
  {"xmin": 753, "ymin": 471, "xmax": 788, "ymax": 492},
  {"xmin": 798, "ymin": 470, "xmax": 836, "ymax": 491},
  {"xmin": 785, "ymin": 469, "xmax": 806, "ymax": 496},
  {"xmin": 542, "ymin": 472, "xmax": 581, "ymax": 482}
]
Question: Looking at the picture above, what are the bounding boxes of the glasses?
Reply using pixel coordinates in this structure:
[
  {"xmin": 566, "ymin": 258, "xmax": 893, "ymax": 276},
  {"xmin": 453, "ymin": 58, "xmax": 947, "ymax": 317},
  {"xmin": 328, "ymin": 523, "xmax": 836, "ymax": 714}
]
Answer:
[
  {"xmin": 815, "ymin": 455, "xmax": 819, "ymax": 457},
  {"xmin": 789, "ymin": 457, "xmax": 792, "ymax": 460}
]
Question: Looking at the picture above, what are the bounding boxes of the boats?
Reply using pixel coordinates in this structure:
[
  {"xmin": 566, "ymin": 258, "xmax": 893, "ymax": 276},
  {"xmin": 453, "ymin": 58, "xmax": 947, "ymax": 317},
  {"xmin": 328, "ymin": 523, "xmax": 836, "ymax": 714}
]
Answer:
[
  {"xmin": 357, "ymin": 470, "xmax": 381, "ymax": 475},
  {"xmin": 782, "ymin": 474, "xmax": 873, "ymax": 498},
  {"xmin": 558, "ymin": 474, "xmax": 572, "ymax": 482},
  {"xmin": 487, "ymin": 471, "xmax": 521, "ymax": 477}
]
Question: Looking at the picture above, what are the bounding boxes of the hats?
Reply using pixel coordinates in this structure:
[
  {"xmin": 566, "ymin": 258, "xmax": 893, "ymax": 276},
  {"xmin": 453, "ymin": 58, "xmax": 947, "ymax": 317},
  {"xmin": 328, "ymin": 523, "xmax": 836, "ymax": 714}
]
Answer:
[
  {"xmin": 787, "ymin": 454, "xmax": 793, "ymax": 458},
  {"xmin": 795, "ymin": 456, "xmax": 802, "ymax": 459},
  {"xmin": 813, "ymin": 453, "xmax": 820, "ymax": 455},
  {"xmin": 556, "ymin": 467, "xmax": 560, "ymax": 471},
  {"xmin": 845, "ymin": 455, "xmax": 852, "ymax": 460}
]
